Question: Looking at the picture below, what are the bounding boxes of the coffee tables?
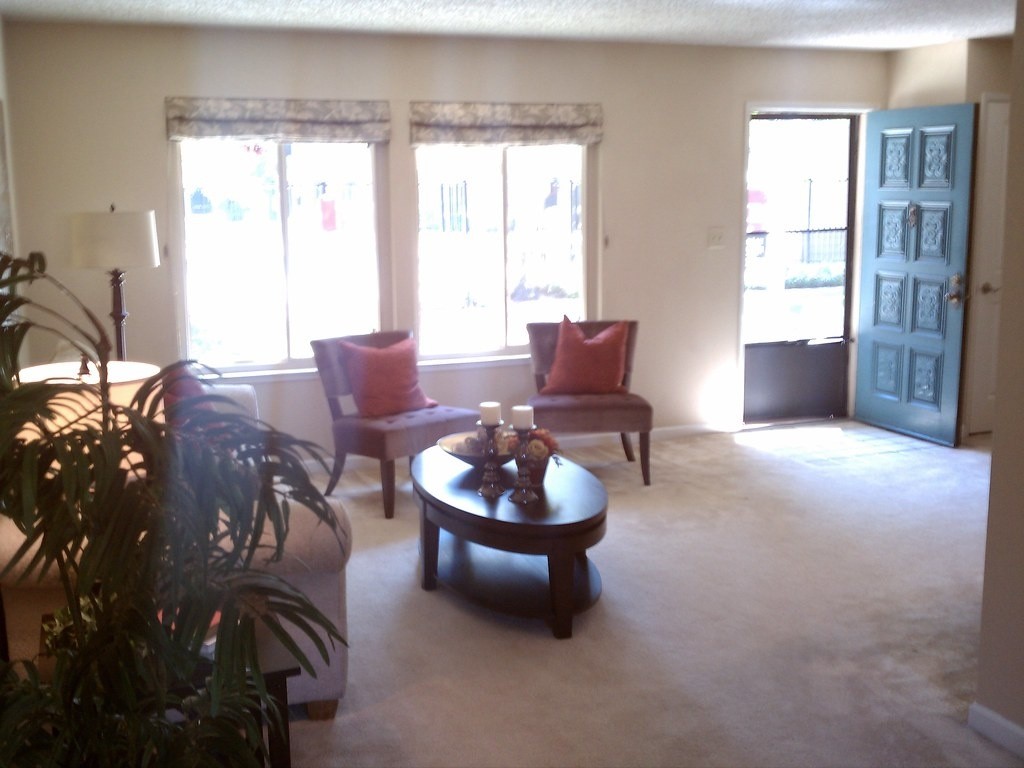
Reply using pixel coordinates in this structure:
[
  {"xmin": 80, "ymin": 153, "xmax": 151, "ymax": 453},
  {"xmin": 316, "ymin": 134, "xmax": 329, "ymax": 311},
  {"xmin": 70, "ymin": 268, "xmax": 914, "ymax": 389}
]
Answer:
[{"xmin": 410, "ymin": 445, "xmax": 607, "ymax": 639}]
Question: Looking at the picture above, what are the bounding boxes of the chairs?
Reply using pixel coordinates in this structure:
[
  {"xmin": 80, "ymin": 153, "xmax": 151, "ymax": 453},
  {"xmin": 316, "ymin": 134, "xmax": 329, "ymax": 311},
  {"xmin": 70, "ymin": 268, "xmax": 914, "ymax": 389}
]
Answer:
[
  {"xmin": 526, "ymin": 320, "xmax": 652, "ymax": 485},
  {"xmin": 310, "ymin": 329, "xmax": 480, "ymax": 518}
]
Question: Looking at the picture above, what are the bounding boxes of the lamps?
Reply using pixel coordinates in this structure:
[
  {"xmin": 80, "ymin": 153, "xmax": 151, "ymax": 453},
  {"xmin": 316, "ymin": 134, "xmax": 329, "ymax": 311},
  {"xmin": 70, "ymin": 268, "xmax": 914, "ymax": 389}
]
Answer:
[
  {"xmin": 14, "ymin": 362, "xmax": 167, "ymax": 487},
  {"xmin": 64, "ymin": 204, "xmax": 160, "ymax": 361}
]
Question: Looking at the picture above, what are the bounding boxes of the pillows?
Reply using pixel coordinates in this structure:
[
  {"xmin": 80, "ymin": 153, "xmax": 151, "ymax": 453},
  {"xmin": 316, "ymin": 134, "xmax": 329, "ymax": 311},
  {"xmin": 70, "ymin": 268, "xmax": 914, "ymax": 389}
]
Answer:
[
  {"xmin": 538, "ymin": 314, "xmax": 628, "ymax": 394},
  {"xmin": 335, "ymin": 339, "xmax": 438, "ymax": 416}
]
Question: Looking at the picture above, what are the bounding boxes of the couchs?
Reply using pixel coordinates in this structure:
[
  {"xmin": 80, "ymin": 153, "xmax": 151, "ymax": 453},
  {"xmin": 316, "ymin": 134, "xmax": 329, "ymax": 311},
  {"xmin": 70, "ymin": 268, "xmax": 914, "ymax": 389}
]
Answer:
[{"xmin": 0, "ymin": 384, "xmax": 351, "ymax": 721}]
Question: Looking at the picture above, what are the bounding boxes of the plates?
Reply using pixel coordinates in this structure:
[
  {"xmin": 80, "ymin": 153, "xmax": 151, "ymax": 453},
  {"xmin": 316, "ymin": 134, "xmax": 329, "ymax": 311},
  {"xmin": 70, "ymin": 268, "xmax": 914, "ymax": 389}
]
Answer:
[{"xmin": 437, "ymin": 432, "xmax": 516, "ymax": 468}]
